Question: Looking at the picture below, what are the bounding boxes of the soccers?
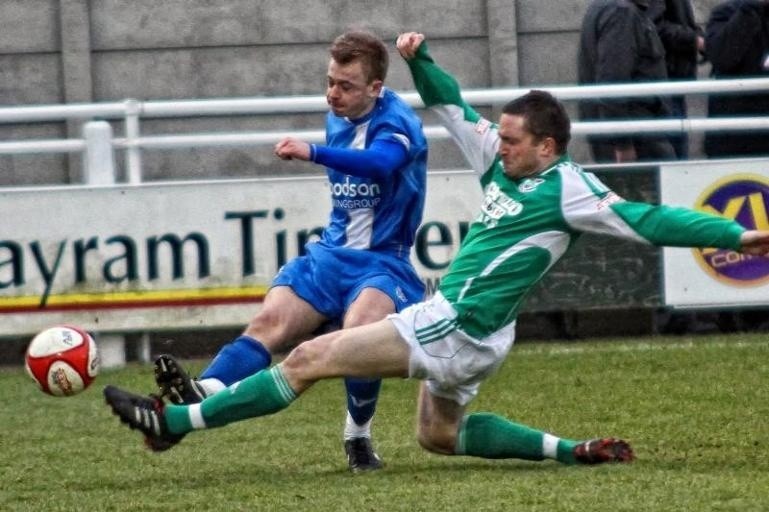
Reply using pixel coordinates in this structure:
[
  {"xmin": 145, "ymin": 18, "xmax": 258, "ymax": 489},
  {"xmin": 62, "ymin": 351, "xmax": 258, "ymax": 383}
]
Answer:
[{"xmin": 25, "ymin": 325, "xmax": 100, "ymax": 396}]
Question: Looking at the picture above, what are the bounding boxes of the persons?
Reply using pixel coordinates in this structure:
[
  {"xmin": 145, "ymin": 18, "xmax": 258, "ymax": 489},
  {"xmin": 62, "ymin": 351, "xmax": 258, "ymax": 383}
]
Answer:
[
  {"xmin": 151, "ymin": 33, "xmax": 427, "ymax": 472},
  {"xmin": 104, "ymin": 32, "xmax": 769, "ymax": 467},
  {"xmin": 570, "ymin": 0, "xmax": 769, "ymax": 162}
]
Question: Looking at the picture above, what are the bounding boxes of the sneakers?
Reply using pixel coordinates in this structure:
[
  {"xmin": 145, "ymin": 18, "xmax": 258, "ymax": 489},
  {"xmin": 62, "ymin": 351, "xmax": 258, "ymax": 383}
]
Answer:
[
  {"xmin": 346, "ymin": 434, "xmax": 384, "ymax": 473},
  {"xmin": 573, "ymin": 436, "xmax": 634, "ymax": 465},
  {"xmin": 103, "ymin": 381, "xmax": 184, "ymax": 453},
  {"xmin": 153, "ymin": 352, "xmax": 202, "ymax": 406}
]
